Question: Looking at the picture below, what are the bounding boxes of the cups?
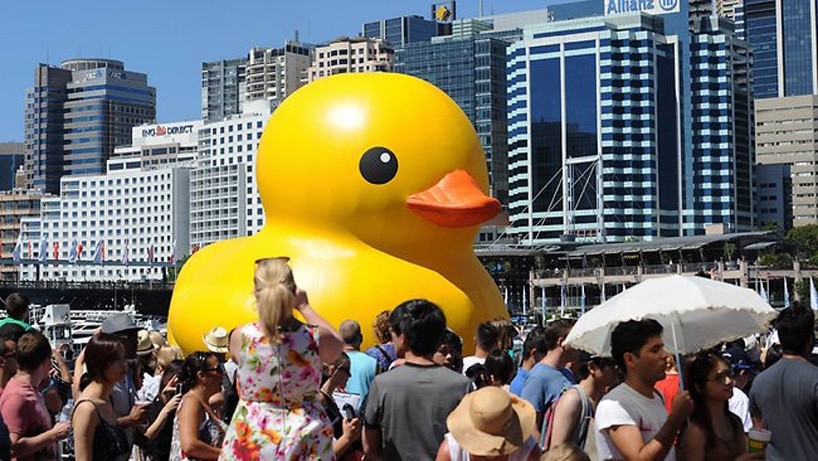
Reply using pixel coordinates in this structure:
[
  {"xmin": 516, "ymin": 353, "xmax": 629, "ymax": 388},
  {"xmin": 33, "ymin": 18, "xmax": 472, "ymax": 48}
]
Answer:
[{"xmin": 748, "ymin": 428, "xmax": 772, "ymax": 453}]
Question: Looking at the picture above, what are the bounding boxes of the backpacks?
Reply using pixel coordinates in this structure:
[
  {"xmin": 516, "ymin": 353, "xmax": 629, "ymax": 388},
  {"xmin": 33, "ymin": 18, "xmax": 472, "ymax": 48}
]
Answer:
[{"xmin": 539, "ymin": 384, "xmax": 588, "ymax": 451}]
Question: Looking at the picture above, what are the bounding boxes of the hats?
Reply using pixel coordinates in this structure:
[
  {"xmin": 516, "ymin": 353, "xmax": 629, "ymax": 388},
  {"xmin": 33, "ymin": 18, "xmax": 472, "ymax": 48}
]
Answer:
[
  {"xmin": 135, "ymin": 330, "xmax": 158, "ymax": 355},
  {"xmin": 203, "ymin": 326, "xmax": 229, "ymax": 352},
  {"xmin": 101, "ymin": 313, "xmax": 144, "ymax": 334},
  {"xmin": 724, "ymin": 347, "xmax": 757, "ymax": 372},
  {"xmin": 447, "ymin": 386, "xmax": 536, "ymax": 456},
  {"xmin": 148, "ymin": 331, "xmax": 165, "ymax": 346}
]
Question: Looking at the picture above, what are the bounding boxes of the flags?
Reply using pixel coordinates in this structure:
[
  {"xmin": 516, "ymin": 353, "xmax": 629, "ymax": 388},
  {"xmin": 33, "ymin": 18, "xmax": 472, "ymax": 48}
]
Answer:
[{"xmin": 12, "ymin": 240, "xmax": 176, "ymax": 265}]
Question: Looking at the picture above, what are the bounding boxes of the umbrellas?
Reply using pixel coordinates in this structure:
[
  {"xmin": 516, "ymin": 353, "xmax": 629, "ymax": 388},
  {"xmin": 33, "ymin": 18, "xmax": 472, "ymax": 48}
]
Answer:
[{"xmin": 561, "ymin": 274, "xmax": 779, "ymax": 428}]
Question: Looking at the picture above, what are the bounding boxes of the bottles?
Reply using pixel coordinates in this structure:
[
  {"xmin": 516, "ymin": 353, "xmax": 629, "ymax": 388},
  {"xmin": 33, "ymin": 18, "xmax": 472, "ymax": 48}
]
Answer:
[{"xmin": 59, "ymin": 398, "xmax": 75, "ymax": 424}]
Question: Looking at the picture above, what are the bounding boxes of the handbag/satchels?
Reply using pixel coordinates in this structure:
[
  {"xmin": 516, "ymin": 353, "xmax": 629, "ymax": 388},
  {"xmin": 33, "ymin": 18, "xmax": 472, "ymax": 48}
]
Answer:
[{"xmin": 180, "ymin": 394, "xmax": 226, "ymax": 461}]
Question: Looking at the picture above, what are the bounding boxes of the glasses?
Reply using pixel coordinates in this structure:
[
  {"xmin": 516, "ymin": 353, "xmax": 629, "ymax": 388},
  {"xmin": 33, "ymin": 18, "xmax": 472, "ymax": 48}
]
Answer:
[
  {"xmin": 202, "ymin": 366, "xmax": 222, "ymax": 372},
  {"xmin": 706, "ymin": 369, "xmax": 734, "ymax": 383},
  {"xmin": 253, "ymin": 256, "xmax": 291, "ymax": 274}
]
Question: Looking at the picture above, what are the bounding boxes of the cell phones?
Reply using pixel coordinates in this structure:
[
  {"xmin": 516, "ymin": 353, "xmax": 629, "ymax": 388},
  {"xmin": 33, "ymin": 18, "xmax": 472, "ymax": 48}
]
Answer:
[{"xmin": 175, "ymin": 383, "xmax": 182, "ymax": 394}]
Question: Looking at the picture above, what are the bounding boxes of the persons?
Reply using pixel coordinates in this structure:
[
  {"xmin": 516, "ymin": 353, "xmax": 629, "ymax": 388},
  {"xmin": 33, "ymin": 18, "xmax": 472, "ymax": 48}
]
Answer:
[
  {"xmin": 594, "ymin": 318, "xmax": 695, "ymax": 461},
  {"xmin": 0, "ymin": 293, "xmax": 240, "ymax": 461},
  {"xmin": 218, "ymin": 259, "xmax": 343, "ymax": 461},
  {"xmin": 318, "ymin": 299, "xmax": 621, "ymax": 461},
  {"xmin": 666, "ymin": 304, "xmax": 818, "ymax": 461},
  {"xmin": 677, "ymin": 357, "xmax": 765, "ymax": 461}
]
